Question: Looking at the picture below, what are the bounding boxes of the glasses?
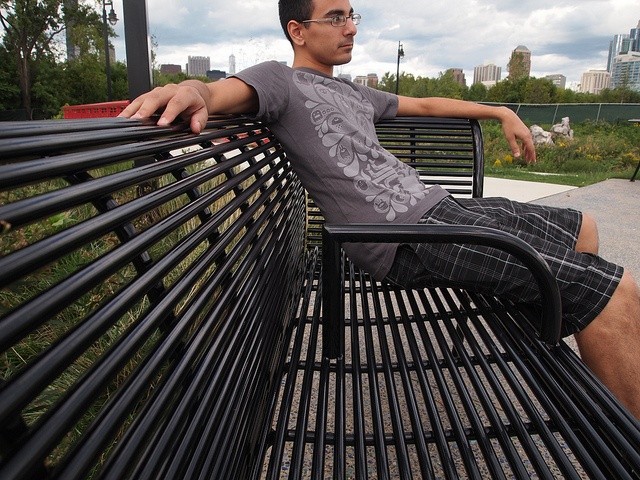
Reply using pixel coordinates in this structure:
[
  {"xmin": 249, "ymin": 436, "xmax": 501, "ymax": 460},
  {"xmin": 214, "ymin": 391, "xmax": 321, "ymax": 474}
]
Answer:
[{"xmin": 297, "ymin": 13, "xmax": 363, "ymax": 27}]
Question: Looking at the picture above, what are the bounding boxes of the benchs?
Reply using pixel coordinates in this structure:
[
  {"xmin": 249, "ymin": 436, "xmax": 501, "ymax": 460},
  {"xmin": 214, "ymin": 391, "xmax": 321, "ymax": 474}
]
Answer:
[{"xmin": 1, "ymin": 109, "xmax": 640, "ymax": 480}]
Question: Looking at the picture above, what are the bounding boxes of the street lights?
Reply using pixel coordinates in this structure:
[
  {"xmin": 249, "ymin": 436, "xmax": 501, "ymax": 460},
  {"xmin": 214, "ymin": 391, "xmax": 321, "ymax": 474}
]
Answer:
[
  {"xmin": 103, "ymin": 0, "xmax": 119, "ymax": 102},
  {"xmin": 396, "ymin": 41, "xmax": 404, "ymax": 94}
]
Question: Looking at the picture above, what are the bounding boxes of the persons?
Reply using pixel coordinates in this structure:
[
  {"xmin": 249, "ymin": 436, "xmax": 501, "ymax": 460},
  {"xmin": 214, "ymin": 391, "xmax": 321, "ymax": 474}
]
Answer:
[{"xmin": 116, "ymin": 0, "xmax": 640, "ymax": 421}]
